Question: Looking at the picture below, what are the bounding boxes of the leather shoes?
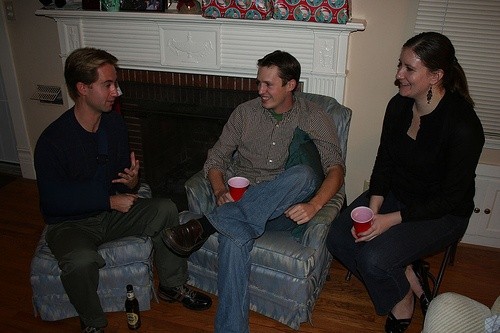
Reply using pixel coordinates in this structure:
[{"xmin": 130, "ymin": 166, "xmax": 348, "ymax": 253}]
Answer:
[{"xmin": 159, "ymin": 218, "xmax": 210, "ymax": 255}]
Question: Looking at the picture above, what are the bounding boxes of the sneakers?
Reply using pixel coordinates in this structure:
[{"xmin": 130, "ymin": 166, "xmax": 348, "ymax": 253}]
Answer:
[
  {"xmin": 79, "ymin": 317, "xmax": 103, "ymax": 333},
  {"xmin": 158, "ymin": 284, "xmax": 212, "ymax": 310}
]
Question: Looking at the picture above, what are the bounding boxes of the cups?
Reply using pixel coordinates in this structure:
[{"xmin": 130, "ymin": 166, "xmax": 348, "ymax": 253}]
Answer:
[
  {"xmin": 351, "ymin": 206, "xmax": 374, "ymax": 238},
  {"xmin": 228, "ymin": 177, "xmax": 250, "ymax": 202}
]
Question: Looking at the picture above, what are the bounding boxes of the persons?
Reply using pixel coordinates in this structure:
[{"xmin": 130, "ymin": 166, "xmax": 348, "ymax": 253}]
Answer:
[
  {"xmin": 33, "ymin": 47, "xmax": 213, "ymax": 333},
  {"xmin": 159, "ymin": 50, "xmax": 346, "ymax": 333},
  {"xmin": 326, "ymin": 32, "xmax": 486, "ymax": 333}
]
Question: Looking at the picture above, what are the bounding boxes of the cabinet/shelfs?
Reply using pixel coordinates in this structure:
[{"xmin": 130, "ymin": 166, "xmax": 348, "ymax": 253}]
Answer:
[{"xmin": 458, "ymin": 165, "xmax": 500, "ymax": 249}]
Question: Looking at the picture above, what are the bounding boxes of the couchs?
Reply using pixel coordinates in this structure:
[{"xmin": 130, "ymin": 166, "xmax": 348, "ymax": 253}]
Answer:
[{"xmin": 179, "ymin": 92, "xmax": 353, "ymax": 331}]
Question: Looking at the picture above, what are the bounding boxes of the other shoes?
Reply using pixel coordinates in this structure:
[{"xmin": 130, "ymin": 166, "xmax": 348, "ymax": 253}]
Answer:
[
  {"xmin": 413, "ymin": 261, "xmax": 431, "ymax": 316},
  {"xmin": 385, "ymin": 293, "xmax": 415, "ymax": 333}
]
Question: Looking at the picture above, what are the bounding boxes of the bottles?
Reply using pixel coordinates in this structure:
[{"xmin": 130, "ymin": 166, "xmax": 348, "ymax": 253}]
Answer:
[{"xmin": 125, "ymin": 285, "xmax": 142, "ymax": 330}]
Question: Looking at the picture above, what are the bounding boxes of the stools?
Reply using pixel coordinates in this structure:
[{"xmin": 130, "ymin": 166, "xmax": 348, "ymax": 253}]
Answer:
[{"xmin": 30, "ymin": 184, "xmax": 159, "ymax": 323}]
